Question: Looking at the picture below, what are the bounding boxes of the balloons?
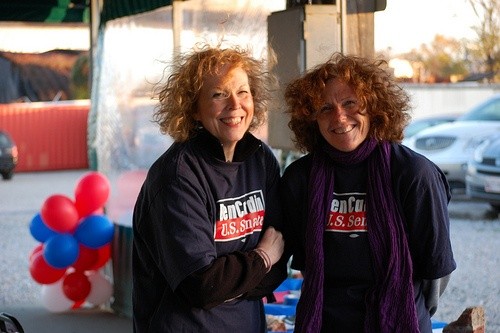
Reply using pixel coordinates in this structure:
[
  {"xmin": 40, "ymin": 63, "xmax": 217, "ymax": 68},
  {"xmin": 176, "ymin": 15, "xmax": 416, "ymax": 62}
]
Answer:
[{"xmin": 29, "ymin": 172, "xmax": 115, "ymax": 311}]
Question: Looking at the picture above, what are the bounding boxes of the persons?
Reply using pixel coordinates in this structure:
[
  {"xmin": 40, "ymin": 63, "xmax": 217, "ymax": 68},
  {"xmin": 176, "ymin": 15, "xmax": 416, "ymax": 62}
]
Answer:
[
  {"xmin": 282, "ymin": 51, "xmax": 457, "ymax": 333},
  {"xmin": 132, "ymin": 44, "xmax": 282, "ymax": 333}
]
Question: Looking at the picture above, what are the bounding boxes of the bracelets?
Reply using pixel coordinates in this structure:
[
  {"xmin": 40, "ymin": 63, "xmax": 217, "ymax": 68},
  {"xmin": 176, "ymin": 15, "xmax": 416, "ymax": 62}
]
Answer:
[{"xmin": 254, "ymin": 248, "xmax": 272, "ymax": 272}]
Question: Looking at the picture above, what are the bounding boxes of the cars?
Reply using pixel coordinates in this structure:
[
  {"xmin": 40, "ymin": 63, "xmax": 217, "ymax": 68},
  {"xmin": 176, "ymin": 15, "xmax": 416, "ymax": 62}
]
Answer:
[
  {"xmin": 403, "ymin": 96, "xmax": 499, "ymax": 208},
  {"xmin": 0, "ymin": 130, "xmax": 19, "ymax": 179}
]
